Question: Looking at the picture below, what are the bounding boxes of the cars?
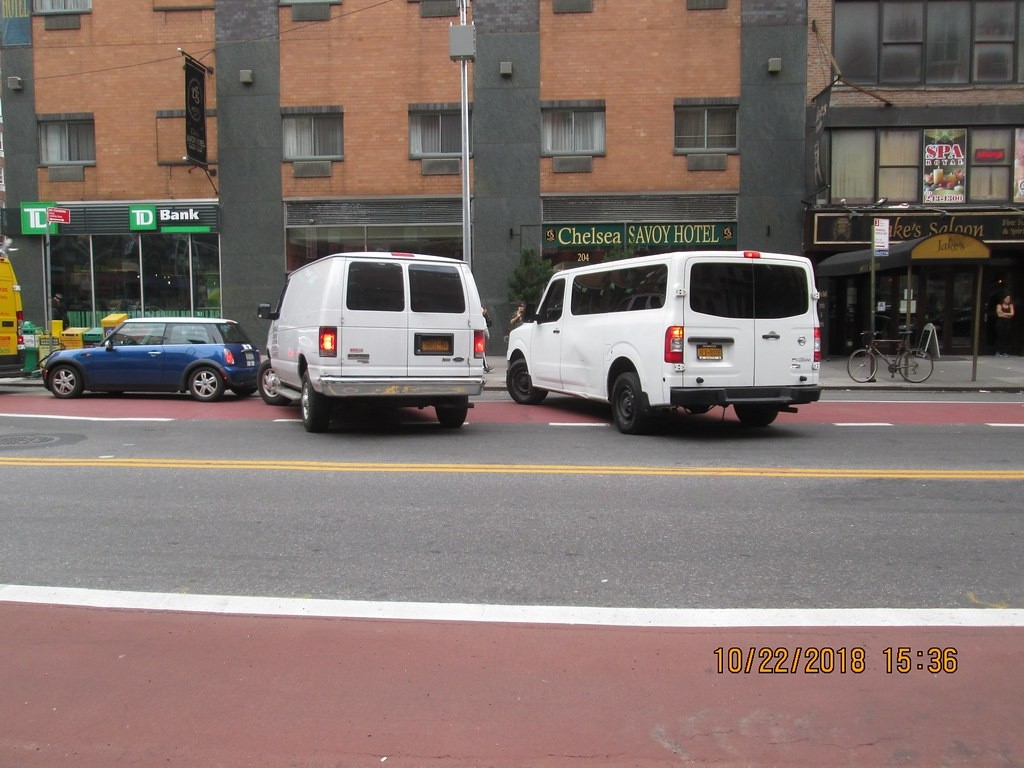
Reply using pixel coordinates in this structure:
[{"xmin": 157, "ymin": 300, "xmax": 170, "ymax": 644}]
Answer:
[{"xmin": 43, "ymin": 317, "xmax": 261, "ymax": 403}]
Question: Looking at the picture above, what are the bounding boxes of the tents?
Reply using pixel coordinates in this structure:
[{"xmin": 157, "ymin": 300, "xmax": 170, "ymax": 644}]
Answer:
[{"xmin": 816, "ymin": 232, "xmax": 991, "ymax": 383}]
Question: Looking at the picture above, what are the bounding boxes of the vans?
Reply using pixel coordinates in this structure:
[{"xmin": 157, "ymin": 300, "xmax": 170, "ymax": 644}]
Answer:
[
  {"xmin": 256, "ymin": 250, "xmax": 485, "ymax": 432},
  {"xmin": 506, "ymin": 249, "xmax": 822, "ymax": 434}
]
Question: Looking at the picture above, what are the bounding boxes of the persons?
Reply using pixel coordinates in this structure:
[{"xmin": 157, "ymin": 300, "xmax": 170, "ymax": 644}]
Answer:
[
  {"xmin": 482, "ymin": 304, "xmax": 494, "ymax": 374},
  {"xmin": 501, "ymin": 303, "xmax": 526, "ymax": 383},
  {"xmin": 993, "ymin": 292, "xmax": 1014, "ymax": 358}
]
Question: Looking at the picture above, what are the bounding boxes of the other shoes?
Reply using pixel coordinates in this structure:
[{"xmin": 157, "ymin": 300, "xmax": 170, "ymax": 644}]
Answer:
[
  {"xmin": 996, "ymin": 353, "xmax": 1007, "ymax": 358},
  {"xmin": 484, "ymin": 367, "xmax": 495, "ymax": 374}
]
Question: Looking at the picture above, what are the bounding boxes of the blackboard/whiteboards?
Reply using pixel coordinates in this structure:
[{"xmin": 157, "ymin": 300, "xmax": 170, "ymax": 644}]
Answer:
[{"xmin": 917, "ymin": 323, "xmax": 939, "ymax": 356}]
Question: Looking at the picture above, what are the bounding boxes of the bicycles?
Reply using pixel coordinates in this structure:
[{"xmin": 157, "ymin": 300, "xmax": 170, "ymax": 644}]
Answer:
[{"xmin": 847, "ymin": 330, "xmax": 934, "ymax": 384}]
